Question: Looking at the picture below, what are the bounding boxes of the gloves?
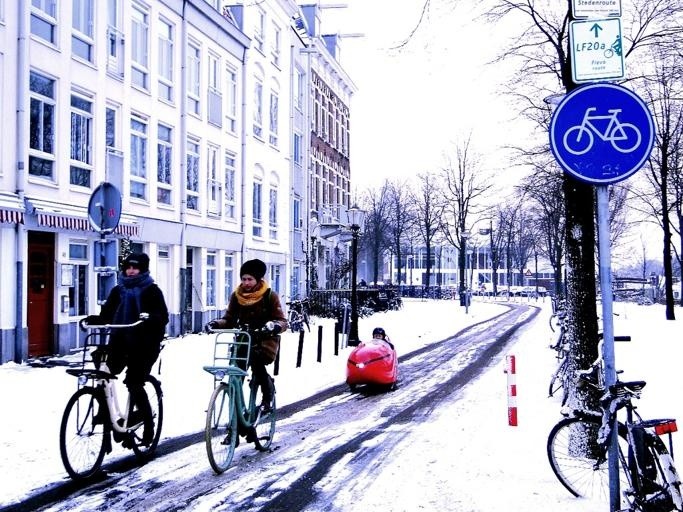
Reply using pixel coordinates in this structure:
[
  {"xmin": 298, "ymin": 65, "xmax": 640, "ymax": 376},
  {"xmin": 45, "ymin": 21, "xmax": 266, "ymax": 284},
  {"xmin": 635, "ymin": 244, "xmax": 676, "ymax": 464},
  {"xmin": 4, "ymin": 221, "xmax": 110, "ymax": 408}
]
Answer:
[
  {"xmin": 205, "ymin": 321, "xmax": 220, "ymax": 333},
  {"xmin": 270, "ymin": 320, "xmax": 282, "ymax": 337}
]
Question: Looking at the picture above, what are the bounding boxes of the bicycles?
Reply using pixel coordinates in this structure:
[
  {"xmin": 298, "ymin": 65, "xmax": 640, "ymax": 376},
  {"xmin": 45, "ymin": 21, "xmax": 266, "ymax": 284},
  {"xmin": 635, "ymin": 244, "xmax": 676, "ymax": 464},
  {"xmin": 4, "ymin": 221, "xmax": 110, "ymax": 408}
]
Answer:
[
  {"xmin": 60, "ymin": 320, "xmax": 162, "ymax": 483},
  {"xmin": 550, "ymin": 293, "xmax": 683, "ymax": 511},
  {"xmin": 282, "ymin": 294, "xmax": 310, "ymax": 333},
  {"xmin": 203, "ymin": 326, "xmax": 277, "ymax": 473}
]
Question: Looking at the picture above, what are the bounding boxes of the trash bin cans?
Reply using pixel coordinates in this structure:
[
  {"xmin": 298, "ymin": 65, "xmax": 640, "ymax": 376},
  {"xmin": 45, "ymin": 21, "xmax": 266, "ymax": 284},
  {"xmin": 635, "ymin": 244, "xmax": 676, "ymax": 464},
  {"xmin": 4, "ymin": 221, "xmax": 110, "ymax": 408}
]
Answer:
[{"xmin": 460, "ymin": 292, "xmax": 471, "ymax": 305}]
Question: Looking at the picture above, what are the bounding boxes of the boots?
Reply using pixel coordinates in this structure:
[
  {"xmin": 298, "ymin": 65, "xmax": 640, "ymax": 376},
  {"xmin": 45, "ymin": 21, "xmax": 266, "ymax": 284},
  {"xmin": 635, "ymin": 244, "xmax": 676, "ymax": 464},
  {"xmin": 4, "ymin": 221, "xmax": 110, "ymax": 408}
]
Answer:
[
  {"xmin": 256, "ymin": 374, "xmax": 275, "ymax": 411},
  {"xmin": 222, "ymin": 432, "xmax": 240, "ymax": 445}
]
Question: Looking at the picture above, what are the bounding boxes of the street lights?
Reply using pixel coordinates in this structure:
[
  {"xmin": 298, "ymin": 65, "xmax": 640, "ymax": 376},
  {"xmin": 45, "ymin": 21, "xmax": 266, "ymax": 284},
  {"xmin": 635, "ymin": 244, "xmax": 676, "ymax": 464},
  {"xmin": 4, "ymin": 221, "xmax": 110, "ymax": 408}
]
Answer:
[{"xmin": 347, "ymin": 201, "xmax": 365, "ymax": 346}]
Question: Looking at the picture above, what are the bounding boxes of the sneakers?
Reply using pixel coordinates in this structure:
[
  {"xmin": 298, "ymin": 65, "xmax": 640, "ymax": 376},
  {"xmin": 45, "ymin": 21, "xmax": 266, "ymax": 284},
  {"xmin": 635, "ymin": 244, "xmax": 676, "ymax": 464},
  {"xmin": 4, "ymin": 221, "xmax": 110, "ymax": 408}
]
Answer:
[{"xmin": 141, "ymin": 420, "xmax": 155, "ymax": 446}]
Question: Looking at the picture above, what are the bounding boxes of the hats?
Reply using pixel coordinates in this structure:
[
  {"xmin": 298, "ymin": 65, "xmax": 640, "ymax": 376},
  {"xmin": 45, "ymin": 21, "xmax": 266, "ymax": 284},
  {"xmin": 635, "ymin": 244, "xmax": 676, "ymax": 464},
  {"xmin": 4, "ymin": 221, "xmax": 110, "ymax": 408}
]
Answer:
[
  {"xmin": 372, "ymin": 328, "xmax": 385, "ymax": 339},
  {"xmin": 240, "ymin": 259, "xmax": 266, "ymax": 283},
  {"xmin": 121, "ymin": 252, "xmax": 150, "ymax": 273}
]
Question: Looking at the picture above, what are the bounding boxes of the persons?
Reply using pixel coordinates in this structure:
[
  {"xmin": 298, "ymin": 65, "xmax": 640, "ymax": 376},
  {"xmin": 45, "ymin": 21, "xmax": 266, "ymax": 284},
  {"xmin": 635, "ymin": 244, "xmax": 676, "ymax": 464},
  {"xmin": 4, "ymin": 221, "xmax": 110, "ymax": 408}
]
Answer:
[
  {"xmin": 78, "ymin": 250, "xmax": 168, "ymax": 446},
  {"xmin": 372, "ymin": 327, "xmax": 393, "ymax": 349},
  {"xmin": 204, "ymin": 257, "xmax": 289, "ymax": 447}
]
